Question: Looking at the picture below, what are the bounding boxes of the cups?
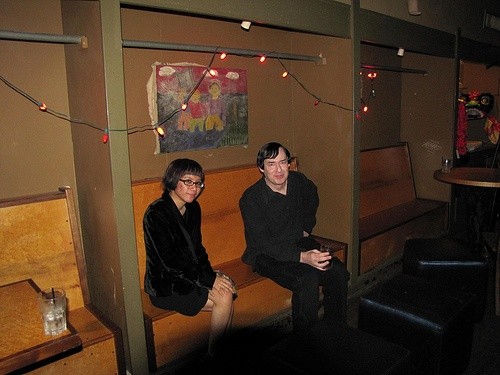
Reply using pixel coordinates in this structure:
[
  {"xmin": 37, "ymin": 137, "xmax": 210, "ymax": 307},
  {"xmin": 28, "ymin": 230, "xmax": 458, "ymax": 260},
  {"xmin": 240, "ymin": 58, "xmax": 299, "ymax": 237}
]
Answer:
[
  {"xmin": 441, "ymin": 157, "xmax": 453, "ymax": 176},
  {"xmin": 37, "ymin": 288, "xmax": 66, "ymax": 336}
]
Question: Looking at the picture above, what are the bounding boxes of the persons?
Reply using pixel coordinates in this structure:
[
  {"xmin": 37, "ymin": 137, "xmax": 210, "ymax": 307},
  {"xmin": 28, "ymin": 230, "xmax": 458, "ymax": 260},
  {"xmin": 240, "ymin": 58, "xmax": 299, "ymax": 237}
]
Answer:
[
  {"xmin": 240, "ymin": 143, "xmax": 348, "ymax": 332},
  {"xmin": 143, "ymin": 158, "xmax": 239, "ymax": 358}
]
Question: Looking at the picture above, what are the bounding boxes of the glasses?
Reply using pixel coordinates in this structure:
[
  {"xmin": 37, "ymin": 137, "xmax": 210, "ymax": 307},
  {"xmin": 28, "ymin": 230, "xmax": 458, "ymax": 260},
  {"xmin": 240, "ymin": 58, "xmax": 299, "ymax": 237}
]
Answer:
[{"xmin": 179, "ymin": 178, "xmax": 204, "ymax": 187}]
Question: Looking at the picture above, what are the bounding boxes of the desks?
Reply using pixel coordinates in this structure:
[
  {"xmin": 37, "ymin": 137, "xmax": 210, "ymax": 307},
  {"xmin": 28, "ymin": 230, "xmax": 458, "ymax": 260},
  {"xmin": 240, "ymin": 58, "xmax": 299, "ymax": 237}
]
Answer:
[
  {"xmin": 0, "ymin": 279, "xmax": 82, "ymax": 375},
  {"xmin": 433, "ymin": 167, "xmax": 500, "ymax": 265}
]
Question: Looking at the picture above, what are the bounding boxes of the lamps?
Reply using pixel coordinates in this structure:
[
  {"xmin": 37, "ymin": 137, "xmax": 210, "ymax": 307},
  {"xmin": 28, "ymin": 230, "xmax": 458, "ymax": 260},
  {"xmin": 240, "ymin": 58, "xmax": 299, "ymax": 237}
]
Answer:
[
  {"xmin": 397, "ymin": 48, "xmax": 404, "ymax": 57},
  {"xmin": 241, "ymin": 20, "xmax": 252, "ymax": 30}
]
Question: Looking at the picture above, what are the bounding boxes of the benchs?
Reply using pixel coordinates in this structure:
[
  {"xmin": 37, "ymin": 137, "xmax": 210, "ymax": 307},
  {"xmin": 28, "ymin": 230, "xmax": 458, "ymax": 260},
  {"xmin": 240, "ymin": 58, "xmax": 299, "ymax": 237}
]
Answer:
[
  {"xmin": 0, "ymin": 185, "xmax": 126, "ymax": 375},
  {"xmin": 130, "ymin": 158, "xmax": 348, "ymax": 372},
  {"xmin": 359, "ymin": 141, "xmax": 449, "ymax": 275}
]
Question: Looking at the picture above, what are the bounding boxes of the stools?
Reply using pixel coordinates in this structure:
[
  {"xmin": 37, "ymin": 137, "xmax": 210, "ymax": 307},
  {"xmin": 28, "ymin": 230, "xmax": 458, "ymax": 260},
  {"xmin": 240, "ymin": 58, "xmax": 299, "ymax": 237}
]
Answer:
[
  {"xmin": 261, "ymin": 318, "xmax": 413, "ymax": 375},
  {"xmin": 403, "ymin": 238, "xmax": 489, "ymax": 323},
  {"xmin": 358, "ymin": 267, "xmax": 476, "ymax": 375}
]
